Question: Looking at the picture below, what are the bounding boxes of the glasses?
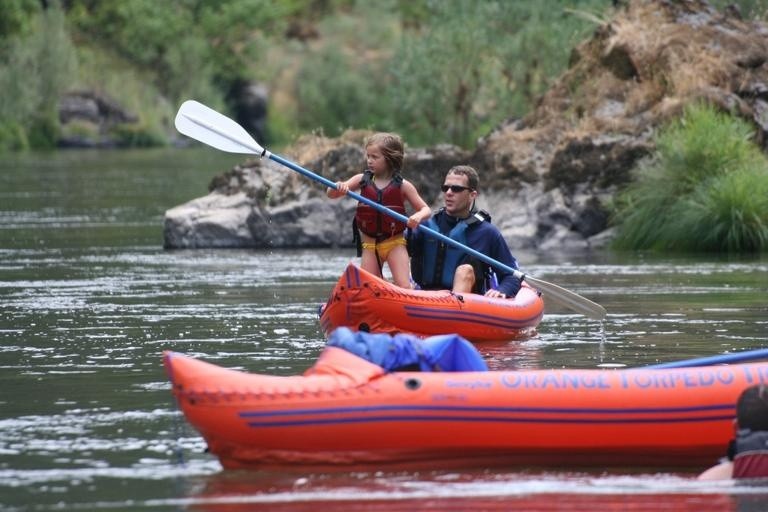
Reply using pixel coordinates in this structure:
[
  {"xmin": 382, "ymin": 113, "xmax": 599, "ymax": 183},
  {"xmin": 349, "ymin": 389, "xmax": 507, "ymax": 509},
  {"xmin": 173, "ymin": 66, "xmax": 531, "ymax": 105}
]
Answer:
[{"xmin": 440, "ymin": 185, "xmax": 470, "ymax": 192}]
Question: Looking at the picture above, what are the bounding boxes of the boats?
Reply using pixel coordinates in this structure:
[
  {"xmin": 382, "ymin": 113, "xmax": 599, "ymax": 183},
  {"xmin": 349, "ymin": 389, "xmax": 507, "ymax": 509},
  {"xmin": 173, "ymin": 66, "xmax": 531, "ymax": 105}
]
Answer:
[
  {"xmin": 159, "ymin": 336, "xmax": 767, "ymax": 475},
  {"xmin": 314, "ymin": 264, "xmax": 549, "ymax": 351}
]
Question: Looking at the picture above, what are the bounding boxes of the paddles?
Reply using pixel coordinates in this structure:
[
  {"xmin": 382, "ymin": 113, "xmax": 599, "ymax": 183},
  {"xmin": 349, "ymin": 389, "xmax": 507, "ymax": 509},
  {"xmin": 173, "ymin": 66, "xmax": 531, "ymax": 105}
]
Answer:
[{"xmin": 175, "ymin": 99, "xmax": 608, "ymax": 321}]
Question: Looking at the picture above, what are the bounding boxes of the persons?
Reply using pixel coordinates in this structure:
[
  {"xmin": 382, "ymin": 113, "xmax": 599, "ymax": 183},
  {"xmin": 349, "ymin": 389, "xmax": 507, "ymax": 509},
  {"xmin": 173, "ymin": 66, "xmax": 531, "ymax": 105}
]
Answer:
[
  {"xmin": 402, "ymin": 163, "xmax": 523, "ymax": 300},
  {"xmin": 695, "ymin": 383, "xmax": 768, "ymax": 483},
  {"xmin": 325, "ymin": 132, "xmax": 433, "ymax": 290}
]
